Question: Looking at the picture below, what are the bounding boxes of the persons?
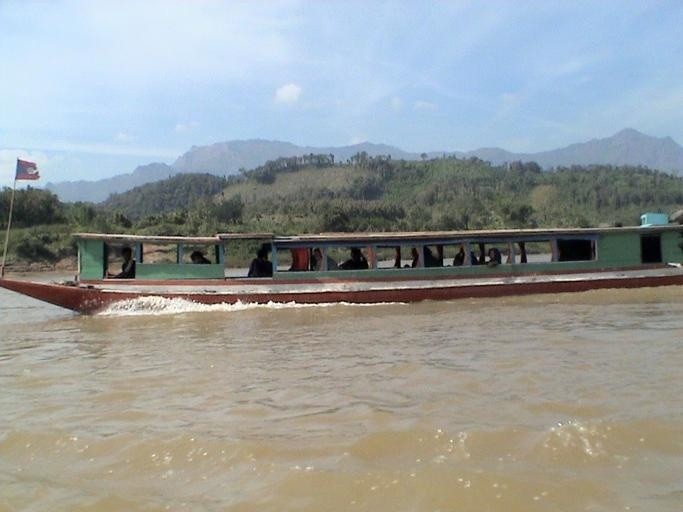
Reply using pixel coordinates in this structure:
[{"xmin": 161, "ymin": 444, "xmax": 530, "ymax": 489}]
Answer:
[
  {"xmin": 191, "ymin": 251, "xmax": 211, "ymax": 264},
  {"xmin": 412, "ymin": 247, "xmax": 501, "ymax": 267},
  {"xmin": 288, "ymin": 247, "xmax": 368, "ymax": 271},
  {"xmin": 120, "ymin": 247, "xmax": 136, "ymax": 278},
  {"xmin": 248, "ymin": 249, "xmax": 272, "ymax": 277}
]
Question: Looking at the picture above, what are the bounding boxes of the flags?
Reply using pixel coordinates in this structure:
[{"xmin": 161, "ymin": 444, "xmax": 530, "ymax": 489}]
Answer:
[{"xmin": 15, "ymin": 159, "xmax": 40, "ymax": 179}]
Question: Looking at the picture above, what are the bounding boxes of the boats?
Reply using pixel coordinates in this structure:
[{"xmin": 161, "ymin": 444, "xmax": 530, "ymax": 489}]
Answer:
[{"xmin": 0, "ymin": 159, "xmax": 682, "ymax": 316}]
